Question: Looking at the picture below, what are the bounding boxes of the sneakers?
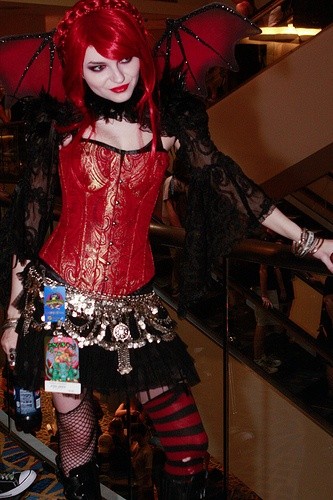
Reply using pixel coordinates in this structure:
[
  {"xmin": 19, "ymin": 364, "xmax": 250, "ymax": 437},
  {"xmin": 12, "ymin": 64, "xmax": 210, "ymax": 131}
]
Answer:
[{"xmin": 0, "ymin": 469, "xmax": 38, "ymax": 499}]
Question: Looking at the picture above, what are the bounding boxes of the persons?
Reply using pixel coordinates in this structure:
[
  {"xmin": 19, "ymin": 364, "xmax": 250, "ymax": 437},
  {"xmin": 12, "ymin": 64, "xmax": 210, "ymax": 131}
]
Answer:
[
  {"xmin": 310, "ymin": 272, "xmax": 333, "ymax": 387},
  {"xmin": 46, "ymin": 395, "xmax": 227, "ymax": 500},
  {"xmin": 161, "ymin": 172, "xmax": 294, "ymax": 367},
  {"xmin": 0, "ymin": 469, "xmax": 37, "ymax": 499},
  {"xmin": 0, "ymin": 0, "xmax": 333, "ymax": 500}
]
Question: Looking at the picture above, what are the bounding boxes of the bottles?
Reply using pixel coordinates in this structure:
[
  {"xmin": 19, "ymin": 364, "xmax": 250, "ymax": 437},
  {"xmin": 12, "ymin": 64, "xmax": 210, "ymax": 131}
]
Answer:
[{"xmin": 13, "ymin": 352, "xmax": 42, "ymax": 434}]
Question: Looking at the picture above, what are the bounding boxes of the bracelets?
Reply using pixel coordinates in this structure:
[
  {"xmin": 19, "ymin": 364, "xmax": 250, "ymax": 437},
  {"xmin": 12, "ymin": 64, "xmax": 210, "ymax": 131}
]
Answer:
[
  {"xmin": 293, "ymin": 227, "xmax": 324, "ymax": 258},
  {"xmin": 1, "ymin": 317, "xmax": 20, "ymax": 329}
]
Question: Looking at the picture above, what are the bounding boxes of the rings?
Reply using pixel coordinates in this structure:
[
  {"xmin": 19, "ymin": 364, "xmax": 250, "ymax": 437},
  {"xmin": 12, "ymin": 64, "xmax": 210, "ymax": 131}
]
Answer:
[{"xmin": 8, "ymin": 347, "xmax": 17, "ymax": 353}]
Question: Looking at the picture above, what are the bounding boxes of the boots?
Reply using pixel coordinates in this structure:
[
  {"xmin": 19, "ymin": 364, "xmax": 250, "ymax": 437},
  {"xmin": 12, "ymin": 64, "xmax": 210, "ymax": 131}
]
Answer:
[
  {"xmin": 55, "ymin": 454, "xmax": 102, "ymax": 500},
  {"xmin": 157, "ymin": 470, "xmax": 208, "ymax": 500}
]
